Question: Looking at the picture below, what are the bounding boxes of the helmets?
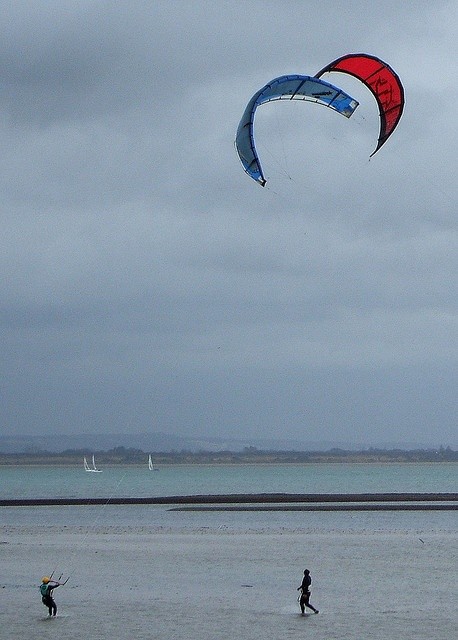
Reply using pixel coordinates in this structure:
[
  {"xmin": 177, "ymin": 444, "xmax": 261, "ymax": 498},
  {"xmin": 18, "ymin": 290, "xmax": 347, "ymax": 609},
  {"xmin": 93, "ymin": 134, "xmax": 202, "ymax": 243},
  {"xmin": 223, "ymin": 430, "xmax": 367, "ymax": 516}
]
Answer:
[{"xmin": 42, "ymin": 577, "xmax": 50, "ymax": 583}]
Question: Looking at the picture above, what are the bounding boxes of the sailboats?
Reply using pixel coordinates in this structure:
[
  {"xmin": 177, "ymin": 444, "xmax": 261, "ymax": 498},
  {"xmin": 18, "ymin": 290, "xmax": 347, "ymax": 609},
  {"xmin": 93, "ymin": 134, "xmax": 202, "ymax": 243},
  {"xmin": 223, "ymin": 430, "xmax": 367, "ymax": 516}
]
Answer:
[
  {"xmin": 148, "ymin": 454, "xmax": 158, "ymax": 470},
  {"xmin": 93, "ymin": 454, "xmax": 102, "ymax": 472},
  {"xmin": 83, "ymin": 456, "xmax": 93, "ymax": 472}
]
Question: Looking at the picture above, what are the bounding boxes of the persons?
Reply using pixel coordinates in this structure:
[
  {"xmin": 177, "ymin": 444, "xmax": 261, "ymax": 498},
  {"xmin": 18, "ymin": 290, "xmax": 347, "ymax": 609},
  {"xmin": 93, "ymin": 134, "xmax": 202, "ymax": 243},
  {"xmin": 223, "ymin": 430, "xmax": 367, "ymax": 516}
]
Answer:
[
  {"xmin": 39, "ymin": 577, "xmax": 61, "ymax": 616},
  {"xmin": 296, "ymin": 569, "xmax": 319, "ymax": 616}
]
